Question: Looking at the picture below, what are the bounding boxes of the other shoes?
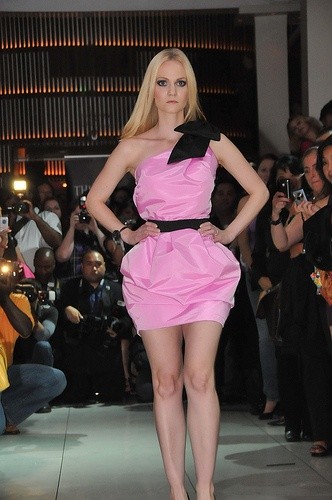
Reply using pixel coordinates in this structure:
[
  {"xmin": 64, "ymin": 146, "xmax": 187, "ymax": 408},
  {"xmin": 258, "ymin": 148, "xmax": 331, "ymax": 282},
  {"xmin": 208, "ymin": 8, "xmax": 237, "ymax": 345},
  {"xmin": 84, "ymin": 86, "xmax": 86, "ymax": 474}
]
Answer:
[
  {"xmin": 35, "ymin": 403, "xmax": 51, "ymax": 413},
  {"xmin": 259, "ymin": 399, "xmax": 332, "ymax": 456},
  {"xmin": 5, "ymin": 429, "xmax": 19, "ymax": 435}
]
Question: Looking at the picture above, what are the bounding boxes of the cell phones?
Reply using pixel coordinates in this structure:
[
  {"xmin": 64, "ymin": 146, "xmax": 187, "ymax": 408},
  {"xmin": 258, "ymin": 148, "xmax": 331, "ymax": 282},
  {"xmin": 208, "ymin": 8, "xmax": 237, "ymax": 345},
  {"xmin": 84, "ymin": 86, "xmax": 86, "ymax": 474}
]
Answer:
[{"xmin": 279, "ymin": 179, "xmax": 290, "ymax": 199}]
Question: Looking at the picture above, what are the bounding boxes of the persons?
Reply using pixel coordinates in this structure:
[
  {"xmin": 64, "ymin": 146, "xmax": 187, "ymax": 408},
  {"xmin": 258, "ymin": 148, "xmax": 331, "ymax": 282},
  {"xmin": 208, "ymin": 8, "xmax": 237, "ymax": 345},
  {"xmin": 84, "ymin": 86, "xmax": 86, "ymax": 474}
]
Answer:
[
  {"xmin": 86, "ymin": 47, "xmax": 270, "ymax": 500},
  {"xmin": 0, "ymin": 101, "xmax": 332, "ymax": 456}
]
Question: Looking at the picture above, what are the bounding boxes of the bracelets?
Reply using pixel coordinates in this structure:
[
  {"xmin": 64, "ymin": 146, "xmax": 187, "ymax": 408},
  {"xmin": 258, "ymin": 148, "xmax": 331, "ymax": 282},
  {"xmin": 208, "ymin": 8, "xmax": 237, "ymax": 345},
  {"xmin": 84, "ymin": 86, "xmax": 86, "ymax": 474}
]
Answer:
[
  {"xmin": 271, "ymin": 216, "xmax": 281, "ymax": 226},
  {"xmin": 112, "ymin": 227, "xmax": 128, "ymax": 239}
]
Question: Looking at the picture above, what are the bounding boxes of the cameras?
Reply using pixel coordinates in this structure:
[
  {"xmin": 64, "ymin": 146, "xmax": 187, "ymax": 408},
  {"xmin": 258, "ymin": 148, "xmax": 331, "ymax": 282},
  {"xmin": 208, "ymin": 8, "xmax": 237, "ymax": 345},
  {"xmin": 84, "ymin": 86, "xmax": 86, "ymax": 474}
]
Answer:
[
  {"xmin": 75, "ymin": 195, "xmax": 89, "ymax": 223},
  {"xmin": 293, "ymin": 188, "xmax": 308, "ymax": 205},
  {"xmin": 25, "ymin": 290, "xmax": 38, "ymax": 301},
  {"xmin": 10, "ymin": 179, "xmax": 28, "ymax": 215}
]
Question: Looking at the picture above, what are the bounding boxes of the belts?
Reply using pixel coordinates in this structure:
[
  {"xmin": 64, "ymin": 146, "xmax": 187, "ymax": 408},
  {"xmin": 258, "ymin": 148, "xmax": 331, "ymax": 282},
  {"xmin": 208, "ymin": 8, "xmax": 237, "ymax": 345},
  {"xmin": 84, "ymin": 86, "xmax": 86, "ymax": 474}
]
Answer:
[{"xmin": 148, "ymin": 217, "xmax": 210, "ymax": 232}]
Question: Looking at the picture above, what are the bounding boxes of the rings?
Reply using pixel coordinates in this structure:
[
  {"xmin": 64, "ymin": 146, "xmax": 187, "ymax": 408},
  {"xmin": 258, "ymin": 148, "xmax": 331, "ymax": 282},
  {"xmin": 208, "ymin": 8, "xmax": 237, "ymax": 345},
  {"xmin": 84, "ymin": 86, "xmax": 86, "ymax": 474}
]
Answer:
[{"xmin": 215, "ymin": 230, "xmax": 218, "ymax": 235}]
